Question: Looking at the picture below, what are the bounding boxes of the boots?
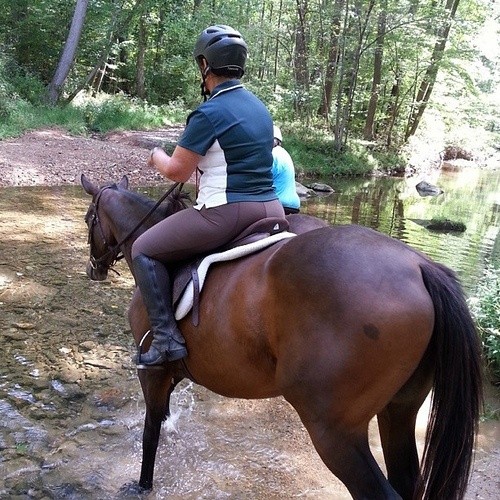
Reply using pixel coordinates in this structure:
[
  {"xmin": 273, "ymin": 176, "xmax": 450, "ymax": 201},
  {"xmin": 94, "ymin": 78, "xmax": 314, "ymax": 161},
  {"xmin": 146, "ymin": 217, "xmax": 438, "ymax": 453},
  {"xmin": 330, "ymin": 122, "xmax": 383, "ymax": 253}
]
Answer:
[{"xmin": 131, "ymin": 254, "xmax": 188, "ymax": 365}]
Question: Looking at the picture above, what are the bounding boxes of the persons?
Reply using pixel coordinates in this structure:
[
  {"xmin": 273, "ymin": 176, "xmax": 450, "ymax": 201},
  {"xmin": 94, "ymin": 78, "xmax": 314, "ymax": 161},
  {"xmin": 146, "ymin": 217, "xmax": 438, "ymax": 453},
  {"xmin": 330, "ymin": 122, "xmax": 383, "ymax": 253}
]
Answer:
[
  {"xmin": 131, "ymin": 25, "xmax": 285, "ymax": 365},
  {"xmin": 271, "ymin": 122, "xmax": 299, "ymax": 214}
]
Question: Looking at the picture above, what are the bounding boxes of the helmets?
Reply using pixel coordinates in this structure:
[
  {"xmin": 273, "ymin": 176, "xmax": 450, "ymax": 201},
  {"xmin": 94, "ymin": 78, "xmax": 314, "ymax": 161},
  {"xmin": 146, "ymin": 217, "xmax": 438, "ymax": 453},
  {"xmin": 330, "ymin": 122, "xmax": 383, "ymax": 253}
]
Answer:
[
  {"xmin": 273, "ymin": 124, "xmax": 283, "ymax": 142},
  {"xmin": 194, "ymin": 24, "xmax": 248, "ymax": 79}
]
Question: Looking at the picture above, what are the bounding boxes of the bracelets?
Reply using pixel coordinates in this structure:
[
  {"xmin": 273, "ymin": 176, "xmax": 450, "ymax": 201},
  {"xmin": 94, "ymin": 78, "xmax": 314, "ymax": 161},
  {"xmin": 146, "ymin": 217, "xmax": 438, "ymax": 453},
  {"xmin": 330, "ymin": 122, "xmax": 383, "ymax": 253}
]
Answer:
[{"xmin": 150, "ymin": 146, "xmax": 159, "ymax": 162}]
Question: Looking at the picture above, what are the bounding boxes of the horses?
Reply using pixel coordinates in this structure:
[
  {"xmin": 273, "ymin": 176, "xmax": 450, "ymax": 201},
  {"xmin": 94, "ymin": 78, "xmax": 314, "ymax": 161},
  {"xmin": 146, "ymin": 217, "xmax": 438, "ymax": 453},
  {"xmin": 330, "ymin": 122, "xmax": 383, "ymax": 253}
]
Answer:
[
  {"xmin": 280, "ymin": 214, "xmax": 329, "ymax": 236},
  {"xmin": 76, "ymin": 170, "xmax": 488, "ymax": 499}
]
